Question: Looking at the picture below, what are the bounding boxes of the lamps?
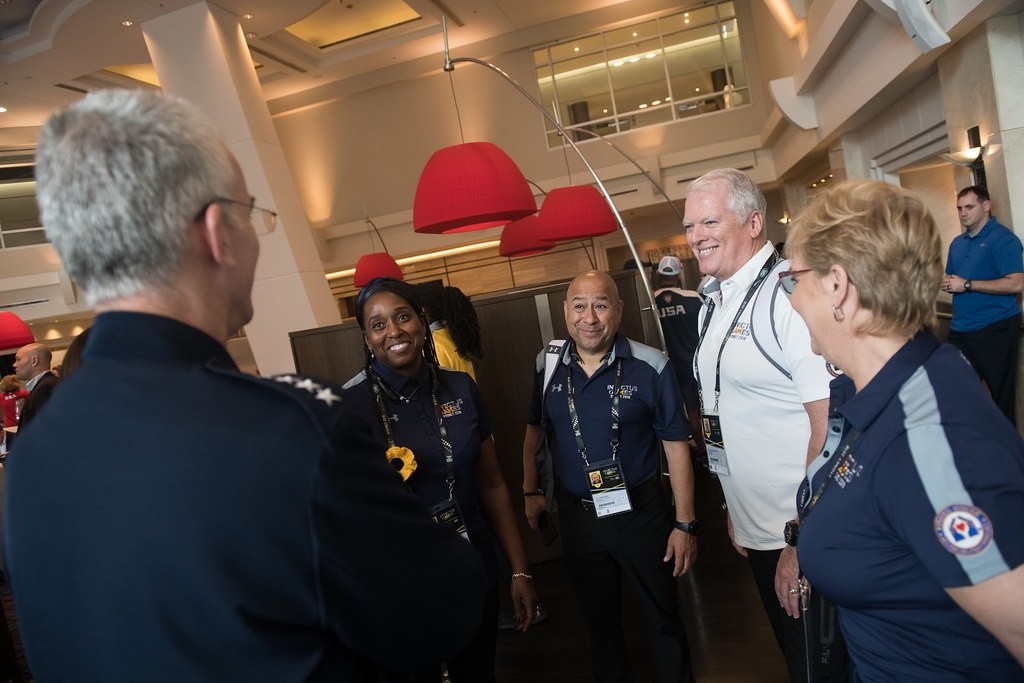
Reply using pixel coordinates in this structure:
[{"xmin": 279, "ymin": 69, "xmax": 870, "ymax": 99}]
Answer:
[
  {"xmin": 406, "ymin": 15, "xmax": 690, "ymax": 421},
  {"xmin": 0, "ymin": 311, "xmax": 36, "ymax": 351},
  {"xmin": 540, "ymin": 98, "xmax": 684, "ymax": 243},
  {"xmin": 938, "ymin": 123, "xmax": 986, "ymax": 203},
  {"xmin": 497, "ymin": 179, "xmax": 597, "ymax": 272},
  {"xmin": 351, "ymin": 214, "xmax": 401, "ymax": 287}
]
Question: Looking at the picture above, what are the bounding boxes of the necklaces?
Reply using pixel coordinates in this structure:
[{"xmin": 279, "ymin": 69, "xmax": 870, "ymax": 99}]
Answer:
[{"xmin": 389, "ymin": 384, "xmax": 421, "ymax": 403}]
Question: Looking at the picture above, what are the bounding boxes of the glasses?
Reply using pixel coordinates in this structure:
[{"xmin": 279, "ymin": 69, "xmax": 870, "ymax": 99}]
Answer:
[
  {"xmin": 779, "ymin": 269, "xmax": 854, "ymax": 294},
  {"xmin": 192, "ymin": 197, "xmax": 277, "ymax": 236}
]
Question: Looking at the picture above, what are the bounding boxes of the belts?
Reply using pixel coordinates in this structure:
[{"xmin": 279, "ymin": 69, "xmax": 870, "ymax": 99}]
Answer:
[{"xmin": 556, "ymin": 482, "xmax": 662, "ymax": 514}]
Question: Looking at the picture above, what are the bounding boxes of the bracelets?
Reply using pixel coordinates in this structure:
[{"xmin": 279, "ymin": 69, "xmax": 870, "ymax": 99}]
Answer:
[
  {"xmin": 524, "ymin": 491, "xmax": 540, "ymax": 496},
  {"xmin": 512, "ymin": 573, "xmax": 533, "ymax": 583}
]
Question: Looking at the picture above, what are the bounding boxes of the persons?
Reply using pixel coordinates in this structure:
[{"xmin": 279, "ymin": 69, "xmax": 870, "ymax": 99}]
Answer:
[
  {"xmin": 13, "ymin": 342, "xmax": 59, "ymax": 438},
  {"xmin": 0, "ymin": 85, "xmax": 487, "ymax": 683},
  {"xmin": 652, "ymin": 256, "xmax": 705, "ymax": 451},
  {"xmin": 417, "ymin": 284, "xmax": 483, "ymax": 383},
  {"xmin": 777, "ymin": 177, "xmax": 1024, "ymax": 683},
  {"xmin": 682, "ymin": 167, "xmax": 843, "ymax": 683},
  {"xmin": 0, "ymin": 375, "xmax": 29, "ymax": 427},
  {"xmin": 940, "ymin": 185, "xmax": 1024, "ymax": 427},
  {"xmin": 523, "ymin": 270, "xmax": 697, "ymax": 683},
  {"xmin": 591, "ymin": 473, "xmax": 601, "ymax": 484},
  {"xmin": 340, "ymin": 277, "xmax": 535, "ymax": 683}
]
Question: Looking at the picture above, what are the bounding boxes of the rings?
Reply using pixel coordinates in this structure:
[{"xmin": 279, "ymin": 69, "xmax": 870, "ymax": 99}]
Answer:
[
  {"xmin": 948, "ymin": 286, "xmax": 950, "ymax": 289},
  {"xmin": 789, "ymin": 588, "xmax": 799, "ymax": 593}
]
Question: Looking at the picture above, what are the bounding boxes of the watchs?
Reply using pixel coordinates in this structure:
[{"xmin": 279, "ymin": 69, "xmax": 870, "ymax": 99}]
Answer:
[
  {"xmin": 784, "ymin": 520, "xmax": 800, "ymax": 547},
  {"xmin": 675, "ymin": 520, "xmax": 698, "ymax": 535},
  {"xmin": 964, "ymin": 280, "xmax": 971, "ymax": 290}
]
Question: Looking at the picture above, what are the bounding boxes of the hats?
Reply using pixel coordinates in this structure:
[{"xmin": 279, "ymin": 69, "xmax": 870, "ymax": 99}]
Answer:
[{"xmin": 658, "ymin": 256, "xmax": 681, "ymax": 275}]
